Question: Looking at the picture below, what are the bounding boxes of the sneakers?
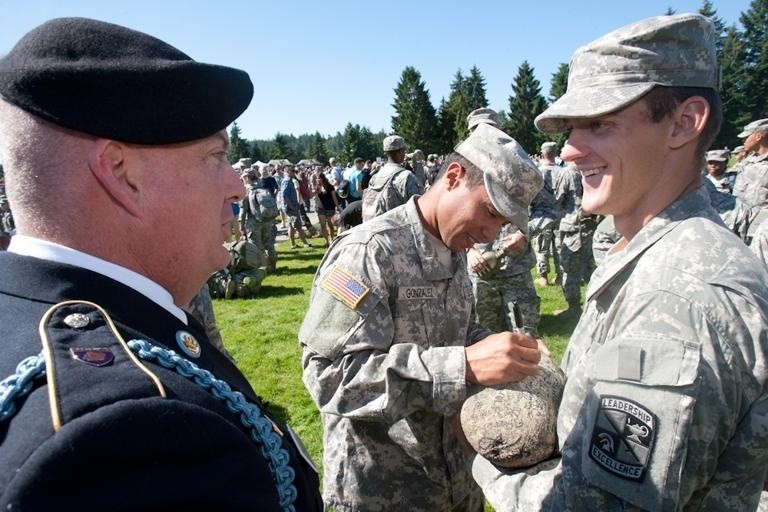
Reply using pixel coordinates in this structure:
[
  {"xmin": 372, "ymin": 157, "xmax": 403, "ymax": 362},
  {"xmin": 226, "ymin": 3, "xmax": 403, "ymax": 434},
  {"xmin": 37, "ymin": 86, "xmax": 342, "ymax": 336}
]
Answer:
[{"xmin": 291, "ymin": 243, "xmax": 312, "ymax": 249}]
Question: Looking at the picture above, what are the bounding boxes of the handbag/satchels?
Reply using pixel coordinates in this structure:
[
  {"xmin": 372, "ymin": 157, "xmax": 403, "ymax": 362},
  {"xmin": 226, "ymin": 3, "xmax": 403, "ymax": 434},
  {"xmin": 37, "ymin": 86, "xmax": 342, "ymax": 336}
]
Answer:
[
  {"xmin": 338, "ymin": 180, "xmax": 350, "ymax": 200},
  {"xmin": 207, "ymin": 241, "xmax": 268, "ymax": 298}
]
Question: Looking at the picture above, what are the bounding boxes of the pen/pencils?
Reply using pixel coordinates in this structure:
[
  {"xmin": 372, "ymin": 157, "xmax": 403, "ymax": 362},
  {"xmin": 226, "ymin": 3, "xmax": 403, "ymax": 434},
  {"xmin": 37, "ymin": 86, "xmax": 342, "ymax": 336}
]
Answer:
[{"xmin": 512, "ymin": 300, "xmax": 525, "ymax": 334}]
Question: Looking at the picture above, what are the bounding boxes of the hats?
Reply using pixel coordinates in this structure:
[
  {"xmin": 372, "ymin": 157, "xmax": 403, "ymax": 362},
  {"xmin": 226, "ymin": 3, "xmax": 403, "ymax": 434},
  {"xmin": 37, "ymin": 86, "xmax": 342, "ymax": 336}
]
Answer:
[
  {"xmin": 707, "ymin": 118, "xmax": 768, "ymax": 162},
  {"xmin": 541, "ymin": 142, "xmax": 557, "ymax": 152},
  {"xmin": 415, "ymin": 150, "xmax": 425, "ymax": 160},
  {"xmin": 237, "ymin": 158, "xmax": 258, "ymax": 178},
  {"xmin": 534, "ymin": 13, "xmax": 721, "ymax": 134},
  {"xmin": 1, "ymin": 18, "xmax": 254, "ymax": 144},
  {"xmin": 383, "ymin": 136, "xmax": 405, "ymax": 152},
  {"xmin": 467, "ymin": 108, "xmax": 499, "ymax": 129},
  {"xmin": 453, "ymin": 123, "xmax": 545, "ymax": 234},
  {"xmin": 330, "ymin": 158, "xmax": 336, "ymax": 163},
  {"xmin": 429, "ymin": 154, "xmax": 434, "ymax": 160}
]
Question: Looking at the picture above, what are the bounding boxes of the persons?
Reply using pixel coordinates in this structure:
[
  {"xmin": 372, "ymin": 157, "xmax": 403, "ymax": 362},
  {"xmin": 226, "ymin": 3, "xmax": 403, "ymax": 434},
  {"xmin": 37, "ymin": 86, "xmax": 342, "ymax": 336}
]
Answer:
[
  {"xmin": 1, "ymin": 172, "xmax": 17, "ymax": 248},
  {"xmin": 297, "ymin": 121, "xmax": 552, "ymax": 512},
  {"xmin": 451, "ymin": 13, "xmax": 768, "ymax": 509},
  {"xmin": 464, "ymin": 107, "xmax": 611, "ymax": 348},
  {"xmin": 206, "ymin": 136, "xmax": 451, "ymax": 310},
  {"xmin": 0, "ymin": 15, "xmax": 323, "ymax": 512},
  {"xmin": 700, "ymin": 114, "xmax": 767, "ymax": 263}
]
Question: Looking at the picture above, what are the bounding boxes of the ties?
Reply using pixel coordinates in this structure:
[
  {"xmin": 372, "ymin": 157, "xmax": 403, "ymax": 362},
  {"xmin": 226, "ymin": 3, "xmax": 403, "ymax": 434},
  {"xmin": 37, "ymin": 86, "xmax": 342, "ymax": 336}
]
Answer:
[{"xmin": 183, "ymin": 309, "xmax": 211, "ymax": 340}]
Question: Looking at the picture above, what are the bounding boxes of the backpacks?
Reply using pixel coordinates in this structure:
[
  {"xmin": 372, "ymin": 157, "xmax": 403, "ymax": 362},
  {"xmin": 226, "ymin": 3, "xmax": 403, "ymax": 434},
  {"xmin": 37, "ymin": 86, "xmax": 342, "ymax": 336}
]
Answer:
[
  {"xmin": 361, "ymin": 170, "xmax": 404, "ymax": 224},
  {"xmin": 245, "ymin": 185, "xmax": 278, "ymax": 222}
]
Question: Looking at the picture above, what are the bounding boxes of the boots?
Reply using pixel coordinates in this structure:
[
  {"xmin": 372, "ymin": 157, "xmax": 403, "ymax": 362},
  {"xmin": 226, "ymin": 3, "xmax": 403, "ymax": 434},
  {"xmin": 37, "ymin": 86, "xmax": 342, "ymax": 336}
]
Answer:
[
  {"xmin": 552, "ymin": 301, "xmax": 582, "ymax": 319},
  {"xmin": 533, "ymin": 274, "xmax": 563, "ymax": 287}
]
